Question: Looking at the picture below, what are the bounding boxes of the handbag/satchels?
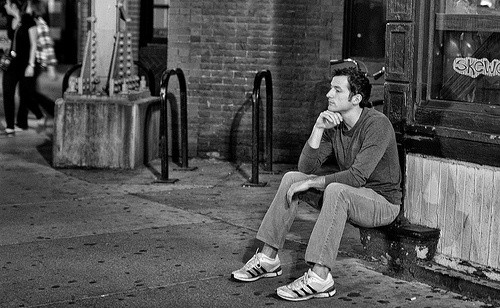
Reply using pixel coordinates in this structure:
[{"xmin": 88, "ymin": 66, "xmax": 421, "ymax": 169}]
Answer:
[{"xmin": 0, "ymin": 55, "xmax": 12, "ymax": 71}]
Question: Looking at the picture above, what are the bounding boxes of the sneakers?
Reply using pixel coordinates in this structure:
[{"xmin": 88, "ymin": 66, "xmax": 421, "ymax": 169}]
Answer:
[
  {"xmin": 275, "ymin": 268, "xmax": 336, "ymax": 301},
  {"xmin": 230, "ymin": 248, "xmax": 282, "ymax": 282}
]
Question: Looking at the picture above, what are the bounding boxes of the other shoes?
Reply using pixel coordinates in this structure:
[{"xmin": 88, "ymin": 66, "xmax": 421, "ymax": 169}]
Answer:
[{"xmin": 0, "ymin": 130, "xmax": 15, "ymax": 137}]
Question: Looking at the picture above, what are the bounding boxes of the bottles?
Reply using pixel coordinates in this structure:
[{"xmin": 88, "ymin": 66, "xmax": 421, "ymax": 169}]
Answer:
[
  {"xmin": 121, "ymin": 78, "xmax": 128, "ymax": 98},
  {"xmin": 140, "ymin": 75, "xmax": 146, "ymax": 90}
]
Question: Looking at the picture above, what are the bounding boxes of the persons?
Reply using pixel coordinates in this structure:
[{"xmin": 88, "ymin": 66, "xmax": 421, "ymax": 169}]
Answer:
[
  {"xmin": 231, "ymin": 66, "xmax": 403, "ymax": 301},
  {"xmin": 0, "ymin": 0, "xmax": 56, "ymax": 139}
]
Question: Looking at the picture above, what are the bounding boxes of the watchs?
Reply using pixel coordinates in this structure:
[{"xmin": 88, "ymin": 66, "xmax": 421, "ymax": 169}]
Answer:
[{"xmin": 28, "ymin": 63, "xmax": 36, "ymax": 68}]
